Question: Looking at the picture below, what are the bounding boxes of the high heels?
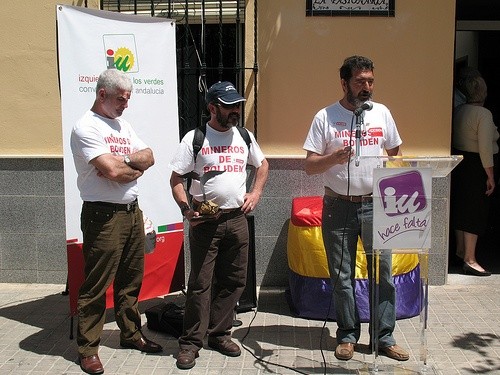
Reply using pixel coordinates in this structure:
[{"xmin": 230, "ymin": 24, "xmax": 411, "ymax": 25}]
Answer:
[
  {"xmin": 461, "ymin": 261, "xmax": 492, "ymax": 276},
  {"xmin": 454, "ymin": 254, "xmax": 464, "ymax": 267}
]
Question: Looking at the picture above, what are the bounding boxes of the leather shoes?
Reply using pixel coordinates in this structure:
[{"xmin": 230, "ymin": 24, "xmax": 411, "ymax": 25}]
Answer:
[
  {"xmin": 120, "ymin": 336, "xmax": 162, "ymax": 353},
  {"xmin": 176, "ymin": 348, "xmax": 199, "ymax": 369},
  {"xmin": 79, "ymin": 354, "xmax": 104, "ymax": 374},
  {"xmin": 208, "ymin": 335, "xmax": 241, "ymax": 357}
]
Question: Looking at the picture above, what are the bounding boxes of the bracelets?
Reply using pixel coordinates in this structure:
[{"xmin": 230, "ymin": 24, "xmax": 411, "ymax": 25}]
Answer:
[{"xmin": 181, "ymin": 208, "xmax": 189, "ymax": 216}]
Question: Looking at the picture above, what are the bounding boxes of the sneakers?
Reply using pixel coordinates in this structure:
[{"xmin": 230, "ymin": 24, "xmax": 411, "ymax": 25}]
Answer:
[
  {"xmin": 368, "ymin": 341, "xmax": 409, "ymax": 361},
  {"xmin": 336, "ymin": 342, "xmax": 354, "ymax": 360}
]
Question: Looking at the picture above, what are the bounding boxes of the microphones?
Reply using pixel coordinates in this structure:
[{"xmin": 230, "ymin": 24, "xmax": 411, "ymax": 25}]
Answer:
[{"xmin": 354, "ymin": 100, "xmax": 373, "ymax": 116}]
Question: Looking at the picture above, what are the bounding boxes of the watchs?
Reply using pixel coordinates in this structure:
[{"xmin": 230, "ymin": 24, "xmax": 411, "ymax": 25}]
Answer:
[{"xmin": 123, "ymin": 155, "xmax": 130, "ymax": 165}]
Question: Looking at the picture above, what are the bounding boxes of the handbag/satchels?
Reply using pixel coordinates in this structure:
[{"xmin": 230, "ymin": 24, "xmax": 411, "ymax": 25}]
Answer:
[{"xmin": 144, "ymin": 302, "xmax": 185, "ymax": 339}]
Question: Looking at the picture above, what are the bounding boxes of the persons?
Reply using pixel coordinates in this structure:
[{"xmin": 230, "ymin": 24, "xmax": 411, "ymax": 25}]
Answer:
[
  {"xmin": 303, "ymin": 56, "xmax": 409, "ymax": 360},
  {"xmin": 453, "ymin": 71, "xmax": 499, "ymax": 276},
  {"xmin": 70, "ymin": 68, "xmax": 163, "ymax": 375},
  {"xmin": 170, "ymin": 81, "xmax": 269, "ymax": 368}
]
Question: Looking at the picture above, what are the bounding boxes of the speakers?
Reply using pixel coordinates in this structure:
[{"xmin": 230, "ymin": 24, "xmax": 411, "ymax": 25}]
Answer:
[{"xmin": 232, "ymin": 214, "xmax": 258, "ymax": 314}]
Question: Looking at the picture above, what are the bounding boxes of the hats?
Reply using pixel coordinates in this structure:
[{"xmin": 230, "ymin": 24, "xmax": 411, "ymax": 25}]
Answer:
[
  {"xmin": 206, "ymin": 81, "xmax": 247, "ymax": 104},
  {"xmin": 465, "ymin": 77, "xmax": 486, "ymax": 102}
]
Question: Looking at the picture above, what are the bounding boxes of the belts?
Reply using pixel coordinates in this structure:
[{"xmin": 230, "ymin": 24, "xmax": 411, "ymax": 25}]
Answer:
[
  {"xmin": 219, "ymin": 208, "xmax": 241, "ymax": 214},
  {"xmin": 88, "ymin": 199, "xmax": 138, "ymax": 212},
  {"xmin": 325, "ymin": 188, "xmax": 373, "ymax": 202}
]
